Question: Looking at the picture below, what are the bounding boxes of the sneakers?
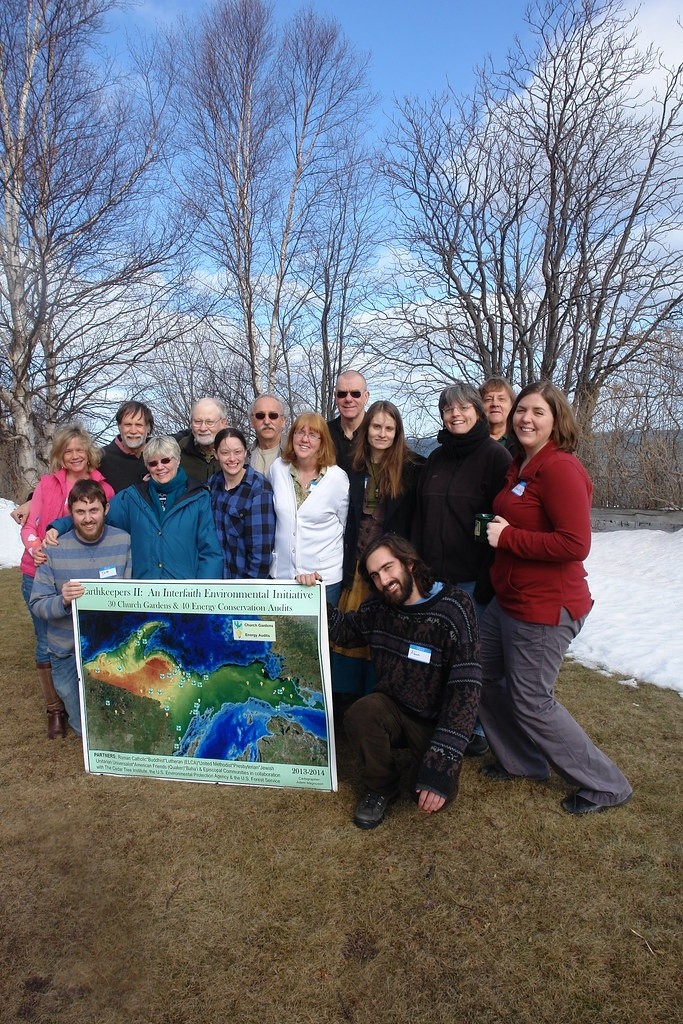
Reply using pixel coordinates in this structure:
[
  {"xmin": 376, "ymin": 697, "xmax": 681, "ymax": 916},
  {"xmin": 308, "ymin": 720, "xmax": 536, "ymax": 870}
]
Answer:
[
  {"xmin": 468, "ymin": 735, "xmax": 490, "ymax": 755},
  {"xmin": 355, "ymin": 786, "xmax": 402, "ymax": 828},
  {"xmin": 561, "ymin": 793, "xmax": 632, "ymax": 814},
  {"xmin": 480, "ymin": 761, "xmax": 550, "ymax": 782}
]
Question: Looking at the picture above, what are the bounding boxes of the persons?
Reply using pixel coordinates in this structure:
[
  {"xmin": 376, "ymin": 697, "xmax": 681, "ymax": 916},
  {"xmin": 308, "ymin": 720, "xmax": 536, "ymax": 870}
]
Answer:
[
  {"xmin": 42, "ymin": 436, "xmax": 223, "ymax": 579},
  {"xmin": 410, "ymin": 382, "xmax": 512, "ymax": 757},
  {"xmin": 29, "ymin": 479, "xmax": 132, "ymax": 736},
  {"xmin": 10, "ymin": 370, "xmax": 517, "ymax": 527},
  {"xmin": 329, "ymin": 400, "xmax": 427, "ymax": 699},
  {"xmin": 295, "ymin": 531, "xmax": 483, "ymax": 828},
  {"xmin": 21, "ymin": 421, "xmax": 115, "ymax": 740},
  {"xmin": 267, "ymin": 412, "xmax": 363, "ymax": 728},
  {"xmin": 477, "ymin": 380, "xmax": 632, "ymax": 814},
  {"xmin": 143, "ymin": 428, "xmax": 276, "ymax": 579}
]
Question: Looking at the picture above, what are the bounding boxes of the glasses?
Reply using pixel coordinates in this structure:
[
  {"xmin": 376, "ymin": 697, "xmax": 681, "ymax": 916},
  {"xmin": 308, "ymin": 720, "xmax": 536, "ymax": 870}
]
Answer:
[
  {"xmin": 336, "ymin": 390, "xmax": 363, "ymax": 398},
  {"xmin": 251, "ymin": 412, "xmax": 282, "ymax": 420},
  {"xmin": 192, "ymin": 418, "xmax": 222, "ymax": 428},
  {"xmin": 148, "ymin": 456, "xmax": 174, "ymax": 467},
  {"xmin": 441, "ymin": 405, "xmax": 474, "ymax": 414},
  {"xmin": 292, "ymin": 429, "xmax": 321, "ymax": 443}
]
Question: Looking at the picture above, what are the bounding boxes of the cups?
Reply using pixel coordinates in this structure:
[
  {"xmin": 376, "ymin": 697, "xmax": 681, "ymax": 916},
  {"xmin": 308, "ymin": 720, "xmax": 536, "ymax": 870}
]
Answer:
[{"xmin": 474, "ymin": 514, "xmax": 497, "ymax": 548}]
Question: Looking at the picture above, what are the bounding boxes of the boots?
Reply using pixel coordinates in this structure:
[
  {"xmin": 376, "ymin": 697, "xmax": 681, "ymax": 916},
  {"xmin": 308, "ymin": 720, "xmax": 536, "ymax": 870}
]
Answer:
[{"xmin": 36, "ymin": 663, "xmax": 67, "ymax": 739}]
